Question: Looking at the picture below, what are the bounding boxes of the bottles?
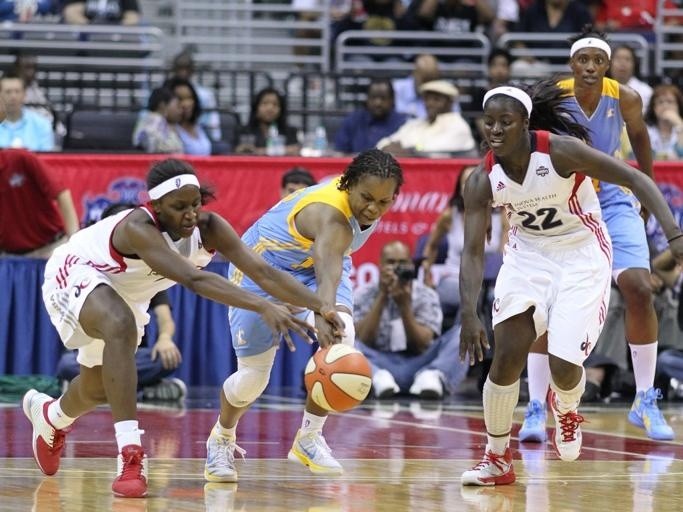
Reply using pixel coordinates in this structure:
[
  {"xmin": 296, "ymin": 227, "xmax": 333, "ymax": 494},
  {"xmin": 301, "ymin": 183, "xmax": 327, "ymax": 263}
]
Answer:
[
  {"xmin": 266, "ymin": 123, "xmax": 279, "ymax": 157},
  {"xmin": 314, "ymin": 124, "xmax": 326, "ymax": 157}
]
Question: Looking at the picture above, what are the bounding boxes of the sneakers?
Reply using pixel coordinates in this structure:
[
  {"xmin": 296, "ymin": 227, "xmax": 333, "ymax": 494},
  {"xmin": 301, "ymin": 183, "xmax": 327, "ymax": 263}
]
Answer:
[
  {"xmin": 204, "ymin": 423, "xmax": 246, "ymax": 483},
  {"xmin": 287, "ymin": 428, "xmax": 344, "ymax": 475},
  {"xmin": 518, "ymin": 399, "xmax": 547, "ymax": 442},
  {"xmin": 461, "ymin": 445, "xmax": 513, "ymax": 487},
  {"xmin": 22, "ymin": 388, "xmax": 74, "ymax": 477},
  {"xmin": 110, "ymin": 444, "xmax": 149, "ymax": 498},
  {"xmin": 628, "ymin": 389, "xmax": 675, "ymax": 440},
  {"xmin": 551, "ymin": 393, "xmax": 583, "ymax": 460}
]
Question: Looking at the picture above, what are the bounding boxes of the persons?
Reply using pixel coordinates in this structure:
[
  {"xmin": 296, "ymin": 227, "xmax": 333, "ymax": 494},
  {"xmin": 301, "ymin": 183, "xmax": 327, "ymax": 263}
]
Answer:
[
  {"xmin": 250, "ymin": 0, "xmax": 683, "ymax": 163},
  {"xmin": 1, "ymin": 146, "xmax": 682, "ymax": 403},
  {"xmin": 519, "ymin": 25, "xmax": 677, "ymax": 443},
  {"xmin": 0, "ymin": 0, "xmax": 304, "ymax": 155},
  {"xmin": 22, "ymin": 157, "xmax": 346, "ymax": 497},
  {"xmin": 204, "ymin": 150, "xmax": 403, "ymax": 482},
  {"xmin": 459, "ymin": 75, "xmax": 683, "ymax": 486}
]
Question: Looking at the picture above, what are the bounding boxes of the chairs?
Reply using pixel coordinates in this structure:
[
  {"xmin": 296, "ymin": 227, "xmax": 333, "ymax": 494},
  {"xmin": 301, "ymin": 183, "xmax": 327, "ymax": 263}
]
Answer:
[
  {"xmin": 198, "ymin": 108, "xmax": 240, "ymax": 155},
  {"xmin": 65, "ymin": 109, "xmax": 136, "ymax": 154}
]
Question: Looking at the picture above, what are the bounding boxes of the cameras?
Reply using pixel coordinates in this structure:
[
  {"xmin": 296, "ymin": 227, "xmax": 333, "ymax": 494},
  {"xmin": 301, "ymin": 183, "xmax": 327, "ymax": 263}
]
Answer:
[{"xmin": 396, "ymin": 265, "xmax": 415, "ymax": 287}]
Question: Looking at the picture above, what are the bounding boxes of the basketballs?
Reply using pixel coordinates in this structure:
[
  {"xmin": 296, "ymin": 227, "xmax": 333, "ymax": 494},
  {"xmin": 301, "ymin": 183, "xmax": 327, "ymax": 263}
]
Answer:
[{"xmin": 304, "ymin": 344, "xmax": 371, "ymax": 413}]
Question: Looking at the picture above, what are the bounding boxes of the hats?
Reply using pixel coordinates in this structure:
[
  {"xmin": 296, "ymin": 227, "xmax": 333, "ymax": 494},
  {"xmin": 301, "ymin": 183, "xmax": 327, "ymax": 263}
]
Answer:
[{"xmin": 418, "ymin": 81, "xmax": 459, "ymax": 102}]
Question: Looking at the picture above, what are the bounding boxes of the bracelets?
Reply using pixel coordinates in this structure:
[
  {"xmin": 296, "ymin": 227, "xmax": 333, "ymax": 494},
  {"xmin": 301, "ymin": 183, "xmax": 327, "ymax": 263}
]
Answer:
[{"xmin": 667, "ymin": 235, "xmax": 683, "ymax": 243}]
